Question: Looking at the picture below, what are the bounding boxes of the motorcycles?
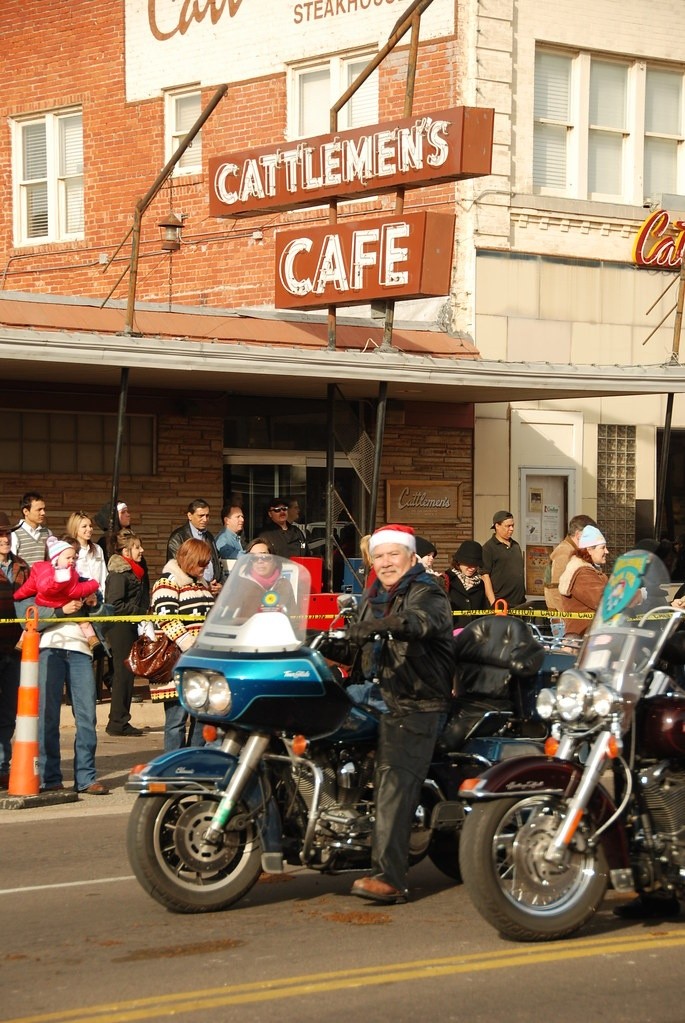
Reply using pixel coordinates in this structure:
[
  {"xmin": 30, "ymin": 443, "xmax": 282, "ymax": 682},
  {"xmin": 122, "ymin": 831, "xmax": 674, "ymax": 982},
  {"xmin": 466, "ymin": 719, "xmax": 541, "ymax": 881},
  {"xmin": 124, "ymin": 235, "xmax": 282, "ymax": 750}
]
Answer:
[{"xmin": 122, "ymin": 550, "xmax": 685, "ymax": 940}]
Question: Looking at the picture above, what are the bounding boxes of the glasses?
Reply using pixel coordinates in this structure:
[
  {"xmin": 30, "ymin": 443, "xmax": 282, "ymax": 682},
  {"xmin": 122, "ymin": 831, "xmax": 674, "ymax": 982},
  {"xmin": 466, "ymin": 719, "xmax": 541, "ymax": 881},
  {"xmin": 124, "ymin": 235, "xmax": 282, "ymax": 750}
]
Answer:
[
  {"xmin": 268, "ymin": 506, "xmax": 289, "ymax": 512},
  {"xmin": 249, "ymin": 555, "xmax": 272, "ymax": 562},
  {"xmin": 198, "ymin": 560, "xmax": 209, "ymax": 567}
]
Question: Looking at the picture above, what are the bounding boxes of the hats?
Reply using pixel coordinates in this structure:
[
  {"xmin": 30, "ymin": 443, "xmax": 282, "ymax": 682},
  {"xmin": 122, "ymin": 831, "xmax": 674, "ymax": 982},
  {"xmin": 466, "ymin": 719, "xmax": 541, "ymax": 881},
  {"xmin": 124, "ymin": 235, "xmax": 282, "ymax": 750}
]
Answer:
[
  {"xmin": 579, "ymin": 525, "xmax": 607, "ymax": 548},
  {"xmin": 415, "ymin": 536, "xmax": 438, "ymax": 559},
  {"xmin": 369, "ymin": 524, "xmax": 417, "ymax": 553},
  {"xmin": 490, "ymin": 510, "xmax": 513, "ymax": 529},
  {"xmin": 46, "ymin": 536, "xmax": 74, "ymax": 560},
  {"xmin": 455, "ymin": 541, "xmax": 484, "ymax": 568},
  {"xmin": 0, "ymin": 511, "xmax": 24, "ymax": 533}
]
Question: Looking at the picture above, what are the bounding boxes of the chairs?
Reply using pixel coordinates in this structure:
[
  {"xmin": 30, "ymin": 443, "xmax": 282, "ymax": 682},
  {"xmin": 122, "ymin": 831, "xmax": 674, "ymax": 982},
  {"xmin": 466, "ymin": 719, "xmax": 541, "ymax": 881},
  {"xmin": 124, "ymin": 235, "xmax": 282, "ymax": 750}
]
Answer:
[{"xmin": 431, "ymin": 612, "xmax": 546, "ymax": 756}]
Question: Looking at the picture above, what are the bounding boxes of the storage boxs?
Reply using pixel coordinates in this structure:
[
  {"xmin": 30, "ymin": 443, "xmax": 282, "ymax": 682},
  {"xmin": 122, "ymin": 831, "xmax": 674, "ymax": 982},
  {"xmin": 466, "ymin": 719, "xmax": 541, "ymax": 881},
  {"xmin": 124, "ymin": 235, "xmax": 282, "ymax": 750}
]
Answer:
[{"xmin": 292, "ymin": 557, "xmax": 345, "ymax": 632}]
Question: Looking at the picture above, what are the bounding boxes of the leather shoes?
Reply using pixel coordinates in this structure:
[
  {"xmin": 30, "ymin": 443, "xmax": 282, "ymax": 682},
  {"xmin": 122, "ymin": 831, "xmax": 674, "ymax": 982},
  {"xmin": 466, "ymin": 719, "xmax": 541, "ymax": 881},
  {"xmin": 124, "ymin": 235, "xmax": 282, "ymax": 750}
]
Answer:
[{"xmin": 350, "ymin": 876, "xmax": 407, "ymax": 903}]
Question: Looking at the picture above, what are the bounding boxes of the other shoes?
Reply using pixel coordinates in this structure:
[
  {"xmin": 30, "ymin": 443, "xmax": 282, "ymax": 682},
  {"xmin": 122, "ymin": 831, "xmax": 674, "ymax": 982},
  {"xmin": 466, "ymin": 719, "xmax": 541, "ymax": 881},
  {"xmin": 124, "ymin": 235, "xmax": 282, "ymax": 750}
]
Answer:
[
  {"xmin": 48, "ymin": 785, "xmax": 66, "ymax": 789},
  {"xmin": 108, "ymin": 725, "xmax": 143, "ymax": 736},
  {"xmin": 612, "ymin": 887, "xmax": 680, "ymax": 919},
  {"xmin": 83, "ymin": 784, "xmax": 107, "ymax": 794}
]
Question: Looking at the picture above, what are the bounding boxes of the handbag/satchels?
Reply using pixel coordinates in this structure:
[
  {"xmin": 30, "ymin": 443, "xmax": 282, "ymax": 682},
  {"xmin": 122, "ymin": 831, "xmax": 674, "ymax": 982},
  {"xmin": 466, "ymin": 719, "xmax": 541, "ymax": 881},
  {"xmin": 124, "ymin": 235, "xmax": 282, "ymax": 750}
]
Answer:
[{"xmin": 130, "ymin": 632, "xmax": 182, "ymax": 683}]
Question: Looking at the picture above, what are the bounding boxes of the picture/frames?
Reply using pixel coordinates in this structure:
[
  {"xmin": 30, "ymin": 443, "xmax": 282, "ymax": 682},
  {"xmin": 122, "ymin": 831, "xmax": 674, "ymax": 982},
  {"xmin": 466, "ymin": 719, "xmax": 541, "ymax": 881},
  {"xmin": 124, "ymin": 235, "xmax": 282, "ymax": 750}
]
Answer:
[{"xmin": 387, "ymin": 480, "xmax": 463, "ymax": 524}]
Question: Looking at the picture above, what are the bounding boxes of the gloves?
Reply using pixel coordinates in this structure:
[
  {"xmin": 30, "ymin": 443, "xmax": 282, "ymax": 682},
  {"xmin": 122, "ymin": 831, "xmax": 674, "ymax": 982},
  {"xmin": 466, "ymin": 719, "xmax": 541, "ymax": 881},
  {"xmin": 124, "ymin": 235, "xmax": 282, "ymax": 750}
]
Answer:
[{"xmin": 343, "ymin": 620, "xmax": 385, "ymax": 645}]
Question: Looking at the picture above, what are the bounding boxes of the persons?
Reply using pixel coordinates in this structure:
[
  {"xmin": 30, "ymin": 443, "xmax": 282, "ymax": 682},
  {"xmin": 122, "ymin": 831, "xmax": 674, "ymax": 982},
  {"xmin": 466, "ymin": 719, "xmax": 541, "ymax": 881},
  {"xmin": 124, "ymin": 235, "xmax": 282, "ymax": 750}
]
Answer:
[
  {"xmin": 0, "ymin": 491, "xmax": 115, "ymax": 794},
  {"xmin": 94, "ymin": 496, "xmax": 685, "ymax": 736},
  {"xmin": 294, "ymin": 525, "xmax": 455, "ymax": 904}
]
